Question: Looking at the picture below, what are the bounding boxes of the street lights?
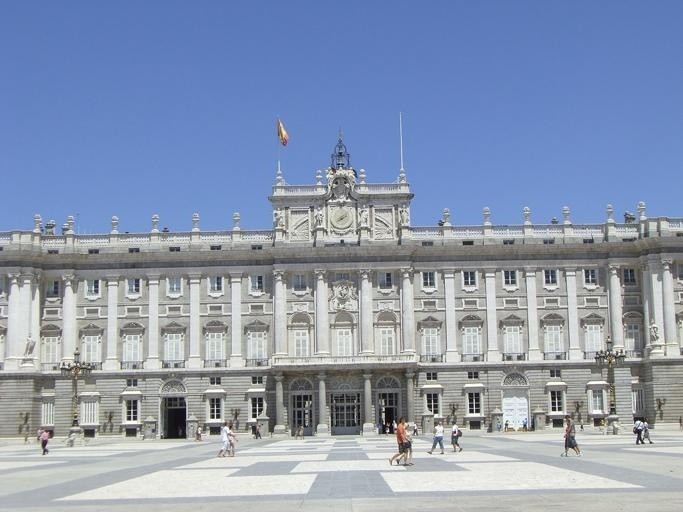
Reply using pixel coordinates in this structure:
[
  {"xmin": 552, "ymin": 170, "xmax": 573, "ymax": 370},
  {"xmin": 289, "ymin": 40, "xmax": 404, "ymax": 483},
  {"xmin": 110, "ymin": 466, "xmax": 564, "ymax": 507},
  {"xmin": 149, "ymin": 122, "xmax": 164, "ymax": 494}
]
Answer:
[
  {"xmin": 60, "ymin": 347, "xmax": 95, "ymax": 428},
  {"xmin": 594, "ymin": 335, "xmax": 625, "ymax": 434}
]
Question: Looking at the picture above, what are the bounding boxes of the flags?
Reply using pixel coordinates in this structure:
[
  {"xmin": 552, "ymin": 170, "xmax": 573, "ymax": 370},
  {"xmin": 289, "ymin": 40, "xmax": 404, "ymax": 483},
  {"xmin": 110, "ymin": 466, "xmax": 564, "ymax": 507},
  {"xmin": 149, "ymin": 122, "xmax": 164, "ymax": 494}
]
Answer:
[{"xmin": 277, "ymin": 118, "xmax": 289, "ymax": 146}]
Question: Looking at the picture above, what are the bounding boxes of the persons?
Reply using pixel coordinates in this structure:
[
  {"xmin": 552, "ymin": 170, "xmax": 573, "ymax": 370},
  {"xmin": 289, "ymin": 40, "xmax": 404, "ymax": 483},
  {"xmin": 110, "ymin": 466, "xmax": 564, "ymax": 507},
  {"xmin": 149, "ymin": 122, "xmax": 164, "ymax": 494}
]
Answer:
[
  {"xmin": 412, "ymin": 423, "xmax": 418, "ymax": 436},
  {"xmin": 643, "ymin": 418, "xmax": 654, "ymax": 444},
  {"xmin": 386, "ymin": 422, "xmax": 390, "ymax": 436},
  {"xmin": 37, "ymin": 427, "xmax": 42, "ymax": 440},
  {"xmin": 635, "ymin": 418, "xmax": 645, "ymax": 445},
  {"xmin": 217, "ymin": 422, "xmax": 229, "ymax": 457},
  {"xmin": 40, "ymin": 430, "xmax": 49, "ymax": 455},
  {"xmin": 451, "ymin": 420, "xmax": 462, "ymax": 452},
  {"xmin": 197, "ymin": 426, "xmax": 201, "ymax": 440},
  {"xmin": 389, "ymin": 417, "xmax": 409, "ymax": 466},
  {"xmin": 426, "ymin": 420, "xmax": 444, "ymax": 454},
  {"xmin": 563, "ymin": 415, "xmax": 581, "ymax": 457},
  {"xmin": 404, "ymin": 423, "xmax": 414, "ymax": 466},
  {"xmin": 228, "ymin": 426, "xmax": 238, "ymax": 457},
  {"xmin": 256, "ymin": 424, "xmax": 262, "ymax": 439},
  {"xmin": 496, "ymin": 417, "xmax": 527, "ymax": 432}
]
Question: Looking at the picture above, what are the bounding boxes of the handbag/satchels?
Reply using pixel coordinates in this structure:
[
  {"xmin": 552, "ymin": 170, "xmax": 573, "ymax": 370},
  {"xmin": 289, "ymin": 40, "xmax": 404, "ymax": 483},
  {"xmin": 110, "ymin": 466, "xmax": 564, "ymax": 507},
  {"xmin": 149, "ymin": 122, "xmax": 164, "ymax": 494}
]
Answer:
[
  {"xmin": 633, "ymin": 427, "xmax": 637, "ymax": 433},
  {"xmin": 452, "ymin": 430, "xmax": 462, "ymax": 436}
]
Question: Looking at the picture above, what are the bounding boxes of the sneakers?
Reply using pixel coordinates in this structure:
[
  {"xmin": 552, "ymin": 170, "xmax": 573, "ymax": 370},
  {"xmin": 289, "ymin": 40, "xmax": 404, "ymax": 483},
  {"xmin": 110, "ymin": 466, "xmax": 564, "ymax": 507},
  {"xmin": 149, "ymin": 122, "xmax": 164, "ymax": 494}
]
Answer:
[
  {"xmin": 561, "ymin": 451, "xmax": 583, "ymax": 457},
  {"xmin": 636, "ymin": 441, "xmax": 654, "ymax": 445},
  {"xmin": 427, "ymin": 447, "xmax": 463, "ymax": 454},
  {"xmin": 389, "ymin": 458, "xmax": 414, "ymax": 466}
]
